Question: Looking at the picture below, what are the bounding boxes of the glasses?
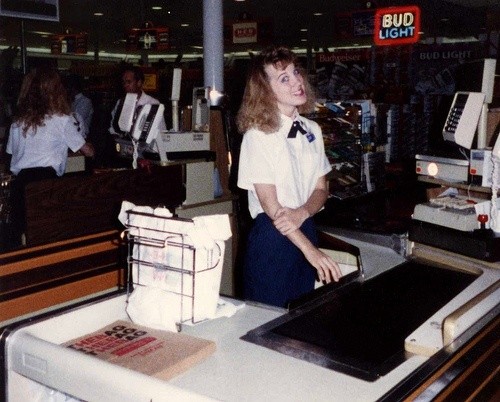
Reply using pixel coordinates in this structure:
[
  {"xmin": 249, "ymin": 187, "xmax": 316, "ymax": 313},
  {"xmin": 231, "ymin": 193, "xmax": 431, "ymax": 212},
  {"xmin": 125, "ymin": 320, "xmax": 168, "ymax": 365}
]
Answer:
[{"xmin": 263, "ymin": 45, "xmax": 289, "ymax": 65}]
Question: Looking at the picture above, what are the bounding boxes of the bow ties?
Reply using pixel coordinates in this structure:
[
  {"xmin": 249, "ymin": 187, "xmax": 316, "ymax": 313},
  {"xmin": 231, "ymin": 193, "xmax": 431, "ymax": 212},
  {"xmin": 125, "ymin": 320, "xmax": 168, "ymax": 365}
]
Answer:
[{"xmin": 287, "ymin": 120, "xmax": 307, "ymax": 138}]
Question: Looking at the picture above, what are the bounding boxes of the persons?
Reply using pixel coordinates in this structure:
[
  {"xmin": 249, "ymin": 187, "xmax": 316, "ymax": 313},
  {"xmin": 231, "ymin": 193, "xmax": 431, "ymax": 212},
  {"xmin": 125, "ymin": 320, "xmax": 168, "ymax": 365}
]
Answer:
[
  {"xmin": 4, "ymin": 65, "xmax": 166, "ymax": 251},
  {"xmin": 232, "ymin": 45, "xmax": 342, "ymax": 306}
]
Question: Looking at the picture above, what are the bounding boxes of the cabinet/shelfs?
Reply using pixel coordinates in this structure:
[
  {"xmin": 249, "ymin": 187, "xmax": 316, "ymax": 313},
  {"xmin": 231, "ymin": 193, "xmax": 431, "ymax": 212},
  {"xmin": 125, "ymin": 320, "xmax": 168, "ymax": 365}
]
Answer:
[{"xmin": 302, "ymin": 101, "xmax": 376, "ymax": 207}]
